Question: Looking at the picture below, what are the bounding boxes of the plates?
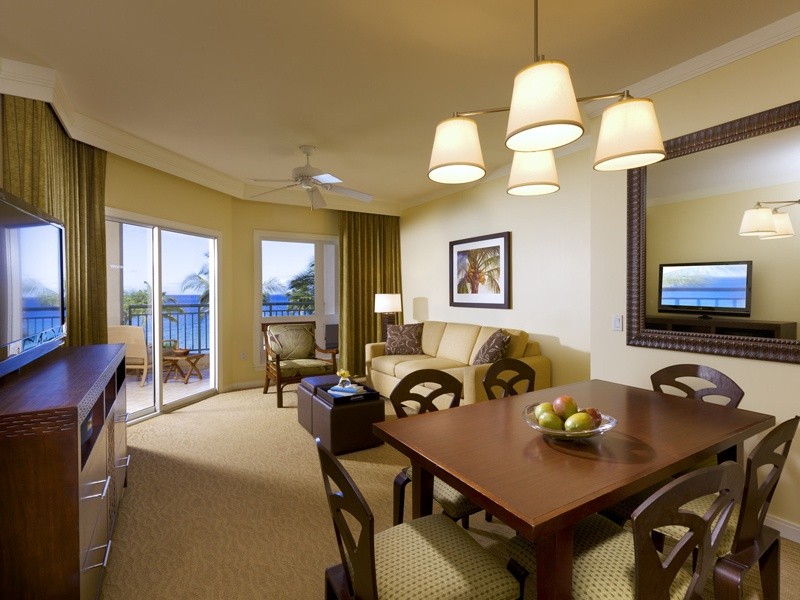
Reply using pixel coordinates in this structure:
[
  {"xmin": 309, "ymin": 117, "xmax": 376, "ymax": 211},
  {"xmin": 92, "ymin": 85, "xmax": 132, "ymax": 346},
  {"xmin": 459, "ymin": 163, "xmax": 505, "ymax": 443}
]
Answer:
[{"xmin": 522, "ymin": 402, "xmax": 617, "ymax": 440}]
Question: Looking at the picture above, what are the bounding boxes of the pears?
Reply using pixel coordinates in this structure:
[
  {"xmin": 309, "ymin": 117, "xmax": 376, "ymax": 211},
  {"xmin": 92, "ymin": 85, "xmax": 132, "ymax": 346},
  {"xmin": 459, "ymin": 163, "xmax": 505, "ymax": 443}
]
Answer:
[{"xmin": 535, "ymin": 396, "xmax": 602, "ymax": 432}]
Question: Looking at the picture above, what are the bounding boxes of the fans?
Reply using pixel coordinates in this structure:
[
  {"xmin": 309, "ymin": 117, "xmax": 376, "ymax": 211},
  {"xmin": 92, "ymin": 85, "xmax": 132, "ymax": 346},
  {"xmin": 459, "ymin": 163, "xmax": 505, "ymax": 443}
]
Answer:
[{"xmin": 248, "ymin": 145, "xmax": 372, "ymax": 212}]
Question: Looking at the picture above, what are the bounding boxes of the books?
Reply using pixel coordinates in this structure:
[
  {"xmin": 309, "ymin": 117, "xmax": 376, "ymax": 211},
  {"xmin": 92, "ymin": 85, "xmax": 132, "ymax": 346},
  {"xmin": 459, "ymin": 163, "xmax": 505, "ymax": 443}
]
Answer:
[{"xmin": 328, "ymin": 384, "xmax": 363, "ymax": 396}]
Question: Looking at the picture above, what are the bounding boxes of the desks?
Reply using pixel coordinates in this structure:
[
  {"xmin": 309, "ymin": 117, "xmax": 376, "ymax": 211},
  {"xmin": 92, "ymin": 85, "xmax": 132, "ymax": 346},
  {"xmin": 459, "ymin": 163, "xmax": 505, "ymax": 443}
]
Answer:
[
  {"xmin": 373, "ymin": 379, "xmax": 776, "ymax": 600},
  {"xmin": 297, "ymin": 376, "xmax": 385, "ymax": 456},
  {"xmin": 163, "ymin": 354, "xmax": 206, "ymax": 384}
]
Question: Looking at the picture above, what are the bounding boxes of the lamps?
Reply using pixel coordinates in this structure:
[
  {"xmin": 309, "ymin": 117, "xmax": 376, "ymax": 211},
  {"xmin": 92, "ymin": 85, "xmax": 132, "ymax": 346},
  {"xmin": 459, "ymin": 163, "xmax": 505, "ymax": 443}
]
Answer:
[
  {"xmin": 427, "ymin": 0, "xmax": 667, "ymax": 197},
  {"xmin": 375, "ymin": 294, "xmax": 402, "ymax": 324},
  {"xmin": 739, "ymin": 199, "xmax": 800, "ymax": 239}
]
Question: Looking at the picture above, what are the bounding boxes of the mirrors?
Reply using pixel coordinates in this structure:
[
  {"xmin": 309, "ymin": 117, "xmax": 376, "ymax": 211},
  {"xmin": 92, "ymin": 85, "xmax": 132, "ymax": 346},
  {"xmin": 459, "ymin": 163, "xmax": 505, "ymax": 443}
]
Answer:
[{"xmin": 626, "ymin": 101, "xmax": 800, "ymax": 365}]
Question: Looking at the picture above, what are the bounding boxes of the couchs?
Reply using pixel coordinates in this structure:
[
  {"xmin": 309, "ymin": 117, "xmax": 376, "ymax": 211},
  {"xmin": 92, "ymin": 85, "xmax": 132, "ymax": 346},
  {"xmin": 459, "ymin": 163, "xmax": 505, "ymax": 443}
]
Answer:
[{"xmin": 365, "ymin": 321, "xmax": 552, "ymax": 413}]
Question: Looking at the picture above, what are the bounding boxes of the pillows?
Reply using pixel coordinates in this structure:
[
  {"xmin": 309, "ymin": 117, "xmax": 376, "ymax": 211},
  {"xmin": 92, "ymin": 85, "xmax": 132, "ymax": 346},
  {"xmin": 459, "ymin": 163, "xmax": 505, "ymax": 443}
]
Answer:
[
  {"xmin": 472, "ymin": 329, "xmax": 511, "ymax": 364},
  {"xmin": 385, "ymin": 323, "xmax": 425, "ymax": 355}
]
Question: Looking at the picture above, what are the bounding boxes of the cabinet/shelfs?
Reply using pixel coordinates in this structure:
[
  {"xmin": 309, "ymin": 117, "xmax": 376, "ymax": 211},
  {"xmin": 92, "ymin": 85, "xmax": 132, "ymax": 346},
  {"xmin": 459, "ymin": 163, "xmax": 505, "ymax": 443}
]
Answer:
[{"xmin": 0, "ymin": 344, "xmax": 131, "ymax": 600}]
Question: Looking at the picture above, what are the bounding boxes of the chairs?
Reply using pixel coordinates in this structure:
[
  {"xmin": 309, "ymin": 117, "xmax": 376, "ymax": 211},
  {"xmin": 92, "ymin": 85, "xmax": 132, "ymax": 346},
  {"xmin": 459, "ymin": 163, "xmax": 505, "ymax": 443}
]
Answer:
[
  {"xmin": 261, "ymin": 321, "xmax": 339, "ymax": 408},
  {"xmin": 162, "ymin": 340, "xmax": 178, "ymax": 379},
  {"xmin": 108, "ymin": 325, "xmax": 148, "ymax": 386},
  {"xmin": 315, "ymin": 364, "xmax": 800, "ymax": 600}
]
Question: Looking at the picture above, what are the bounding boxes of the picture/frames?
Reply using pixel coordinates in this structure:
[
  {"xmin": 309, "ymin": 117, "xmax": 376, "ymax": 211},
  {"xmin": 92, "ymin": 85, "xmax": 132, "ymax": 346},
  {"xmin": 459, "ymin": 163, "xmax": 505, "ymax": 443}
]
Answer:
[{"xmin": 449, "ymin": 232, "xmax": 511, "ymax": 309}]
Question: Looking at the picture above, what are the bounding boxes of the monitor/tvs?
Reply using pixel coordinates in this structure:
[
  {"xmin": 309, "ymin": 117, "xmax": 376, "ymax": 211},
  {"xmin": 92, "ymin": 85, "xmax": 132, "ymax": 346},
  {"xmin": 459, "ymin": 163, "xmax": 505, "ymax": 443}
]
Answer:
[
  {"xmin": 658, "ymin": 259, "xmax": 753, "ymax": 319},
  {"xmin": 0, "ymin": 188, "xmax": 67, "ymax": 380}
]
